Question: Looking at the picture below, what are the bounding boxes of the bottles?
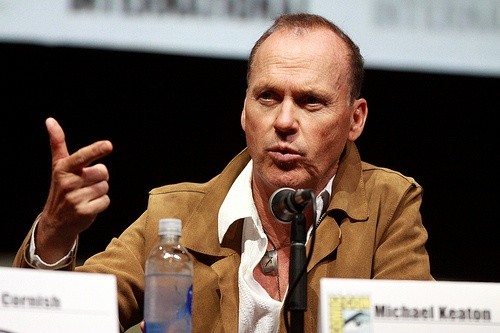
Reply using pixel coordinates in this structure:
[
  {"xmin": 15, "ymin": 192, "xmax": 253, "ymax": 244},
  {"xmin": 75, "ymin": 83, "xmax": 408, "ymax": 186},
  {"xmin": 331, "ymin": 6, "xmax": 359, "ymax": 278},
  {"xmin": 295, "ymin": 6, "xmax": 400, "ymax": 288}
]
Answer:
[{"xmin": 143, "ymin": 218, "xmax": 193, "ymax": 333}]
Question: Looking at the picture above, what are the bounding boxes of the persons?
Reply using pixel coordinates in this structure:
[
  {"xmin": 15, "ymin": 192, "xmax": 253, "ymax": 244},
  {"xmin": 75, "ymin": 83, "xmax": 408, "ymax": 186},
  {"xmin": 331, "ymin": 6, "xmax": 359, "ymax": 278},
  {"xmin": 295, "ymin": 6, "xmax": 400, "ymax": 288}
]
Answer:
[{"xmin": 11, "ymin": 13, "xmax": 438, "ymax": 333}]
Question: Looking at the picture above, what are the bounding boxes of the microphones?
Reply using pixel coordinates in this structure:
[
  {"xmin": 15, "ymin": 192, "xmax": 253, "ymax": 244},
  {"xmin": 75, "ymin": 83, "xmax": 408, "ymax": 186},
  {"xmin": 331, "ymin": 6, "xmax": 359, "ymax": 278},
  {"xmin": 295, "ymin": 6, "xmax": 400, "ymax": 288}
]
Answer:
[{"xmin": 269, "ymin": 186, "xmax": 316, "ymax": 225}]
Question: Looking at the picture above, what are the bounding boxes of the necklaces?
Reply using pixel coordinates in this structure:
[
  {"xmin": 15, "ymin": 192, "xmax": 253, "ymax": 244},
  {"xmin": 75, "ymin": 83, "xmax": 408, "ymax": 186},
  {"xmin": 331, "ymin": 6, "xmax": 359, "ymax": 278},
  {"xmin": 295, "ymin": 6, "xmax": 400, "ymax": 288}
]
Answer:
[{"xmin": 251, "ymin": 180, "xmax": 291, "ymax": 274}]
